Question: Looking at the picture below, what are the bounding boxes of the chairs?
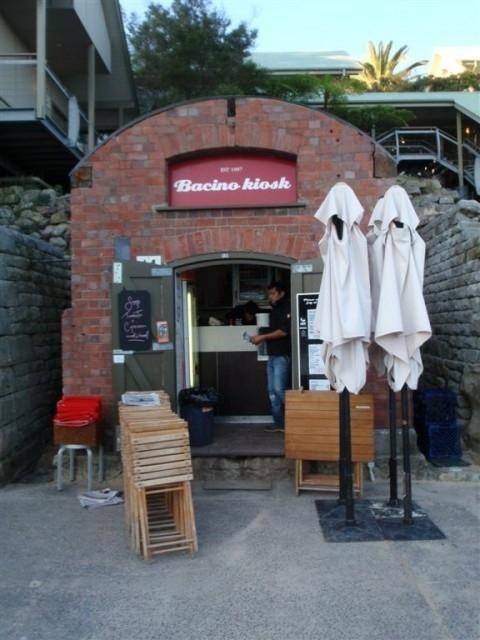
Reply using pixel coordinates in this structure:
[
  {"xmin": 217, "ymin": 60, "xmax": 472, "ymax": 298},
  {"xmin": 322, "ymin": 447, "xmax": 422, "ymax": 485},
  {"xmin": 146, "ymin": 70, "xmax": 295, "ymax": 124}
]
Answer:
[{"xmin": 118, "ymin": 390, "xmax": 199, "ymax": 561}]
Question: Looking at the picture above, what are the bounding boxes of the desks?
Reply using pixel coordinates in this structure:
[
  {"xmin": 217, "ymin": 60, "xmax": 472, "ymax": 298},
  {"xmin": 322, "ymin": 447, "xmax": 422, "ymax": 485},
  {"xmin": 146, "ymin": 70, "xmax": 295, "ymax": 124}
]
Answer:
[{"xmin": 285, "ymin": 389, "xmax": 374, "ymax": 499}]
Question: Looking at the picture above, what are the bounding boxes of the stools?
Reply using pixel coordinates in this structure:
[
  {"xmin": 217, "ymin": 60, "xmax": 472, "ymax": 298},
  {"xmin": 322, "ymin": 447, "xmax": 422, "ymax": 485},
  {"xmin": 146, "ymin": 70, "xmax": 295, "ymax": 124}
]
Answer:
[{"xmin": 57, "ymin": 443, "xmax": 104, "ymax": 491}]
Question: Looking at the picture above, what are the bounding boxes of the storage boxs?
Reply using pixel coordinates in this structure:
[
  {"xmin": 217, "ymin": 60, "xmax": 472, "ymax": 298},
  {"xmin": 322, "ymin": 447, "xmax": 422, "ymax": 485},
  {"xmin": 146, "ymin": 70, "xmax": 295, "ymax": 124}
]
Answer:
[{"xmin": 51, "ymin": 421, "xmax": 104, "ymax": 447}]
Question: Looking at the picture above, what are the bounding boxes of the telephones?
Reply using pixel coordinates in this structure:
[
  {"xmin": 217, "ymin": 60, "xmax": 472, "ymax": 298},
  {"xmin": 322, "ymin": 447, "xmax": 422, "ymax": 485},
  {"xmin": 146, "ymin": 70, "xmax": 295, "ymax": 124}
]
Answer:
[{"xmin": 118, "ymin": 290, "xmax": 151, "ymax": 350}]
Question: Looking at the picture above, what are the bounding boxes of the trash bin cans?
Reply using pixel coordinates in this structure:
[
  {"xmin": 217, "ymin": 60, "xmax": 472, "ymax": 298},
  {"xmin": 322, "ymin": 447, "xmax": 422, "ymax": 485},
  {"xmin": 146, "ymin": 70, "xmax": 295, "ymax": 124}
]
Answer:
[{"xmin": 179, "ymin": 388, "xmax": 215, "ymax": 446}]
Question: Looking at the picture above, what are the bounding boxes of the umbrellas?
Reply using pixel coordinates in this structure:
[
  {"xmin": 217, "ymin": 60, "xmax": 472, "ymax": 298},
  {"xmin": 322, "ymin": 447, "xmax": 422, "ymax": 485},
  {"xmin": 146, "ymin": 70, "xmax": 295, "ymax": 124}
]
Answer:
[
  {"xmin": 372, "ymin": 184, "xmax": 435, "ymax": 523},
  {"xmin": 313, "ymin": 180, "xmax": 372, "ymax": 523},
  {"xmin": 364, "ymin": 197, "xmax": 401, "ymax": 508}
]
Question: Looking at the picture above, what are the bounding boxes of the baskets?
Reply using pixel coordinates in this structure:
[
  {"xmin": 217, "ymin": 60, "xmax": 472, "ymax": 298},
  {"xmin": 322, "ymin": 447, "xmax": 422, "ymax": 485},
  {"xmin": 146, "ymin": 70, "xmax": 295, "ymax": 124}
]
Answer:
[{"xmin": 418, "ymin": 388, "xmax": 461, "ymax": 459}]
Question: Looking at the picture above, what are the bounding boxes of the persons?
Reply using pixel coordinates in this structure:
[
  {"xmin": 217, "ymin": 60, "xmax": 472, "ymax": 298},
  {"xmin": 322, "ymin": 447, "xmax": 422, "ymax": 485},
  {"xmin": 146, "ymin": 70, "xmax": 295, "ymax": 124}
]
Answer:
[
  {"xmin": 248, "ymin": 282, "xmax": 291, "ymax": 433},
  {"xmin": 223, "ymin": 300, "xmax": 262, "ymax": 325}
]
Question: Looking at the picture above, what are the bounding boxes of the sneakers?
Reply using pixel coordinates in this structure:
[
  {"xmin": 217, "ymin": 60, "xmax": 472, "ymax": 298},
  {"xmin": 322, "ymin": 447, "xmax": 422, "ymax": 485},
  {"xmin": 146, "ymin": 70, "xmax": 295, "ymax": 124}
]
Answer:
[{"xmin": 264, "ymin": 424, "xmax": 284, "ymax": 432}]
